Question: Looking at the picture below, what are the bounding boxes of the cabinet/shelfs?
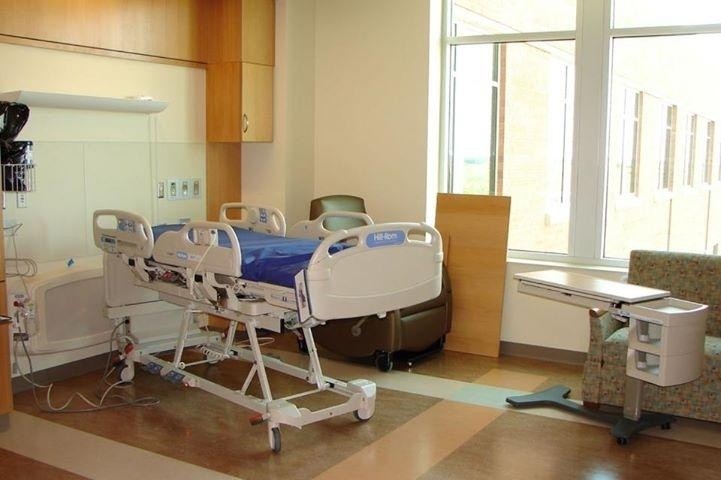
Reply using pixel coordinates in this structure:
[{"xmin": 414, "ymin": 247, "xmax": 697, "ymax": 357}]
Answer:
[{"xmin": 242, "ymin": 63, "xmax": 275, "ymax": 142}]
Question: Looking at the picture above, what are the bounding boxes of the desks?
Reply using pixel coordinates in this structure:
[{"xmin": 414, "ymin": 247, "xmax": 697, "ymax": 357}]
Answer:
[{"xmin": 506, "ymin": 264, "xmax": 708, "ymax": 444}]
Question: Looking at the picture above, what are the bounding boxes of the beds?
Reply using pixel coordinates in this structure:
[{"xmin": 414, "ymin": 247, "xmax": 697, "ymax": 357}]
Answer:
[{"xmin": 93, "ymin": 201, "xmax": 444, "ymax": 453}]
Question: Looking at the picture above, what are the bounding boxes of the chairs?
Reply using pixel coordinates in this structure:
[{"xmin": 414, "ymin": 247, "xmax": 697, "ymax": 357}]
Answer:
[{"xmin": 305, "ymin": 195, "xmax": 452, "ymax": 371}]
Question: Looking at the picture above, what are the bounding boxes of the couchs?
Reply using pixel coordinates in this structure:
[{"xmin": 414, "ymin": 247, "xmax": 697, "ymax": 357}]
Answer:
[{"xmin": 579, "ymin": 250, "xmax": 719, "ymax": 425}]
